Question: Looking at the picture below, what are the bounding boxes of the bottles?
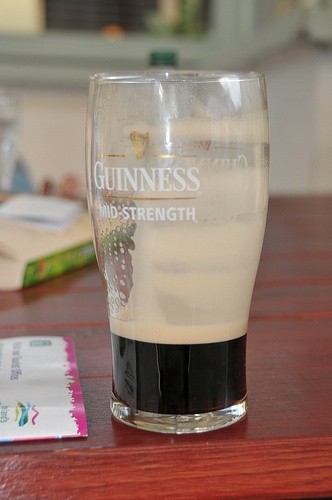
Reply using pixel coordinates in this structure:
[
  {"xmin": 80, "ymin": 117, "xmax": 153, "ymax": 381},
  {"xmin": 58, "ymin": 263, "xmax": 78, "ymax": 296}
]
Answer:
[{"xmin": 146, "ymin": 52, "xmax": 178, "ymax": 72}]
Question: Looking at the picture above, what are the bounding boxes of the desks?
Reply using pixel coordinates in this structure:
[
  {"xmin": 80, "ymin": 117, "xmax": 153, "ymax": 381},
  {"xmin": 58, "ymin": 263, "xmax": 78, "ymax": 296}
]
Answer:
[{"xmin": 0, "ymin": 194, "xmax": 332, "ymax": 500}]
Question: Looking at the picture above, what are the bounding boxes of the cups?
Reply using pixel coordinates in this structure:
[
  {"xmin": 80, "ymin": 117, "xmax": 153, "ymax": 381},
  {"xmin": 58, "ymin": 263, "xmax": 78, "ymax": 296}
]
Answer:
[
  {"xmin": 85, "ymin": 67, "xmax": 271, "ymax": 435},
  {"xmin": 0, "ymin": 85, "xmax": 23, "ymax": 193}
]
